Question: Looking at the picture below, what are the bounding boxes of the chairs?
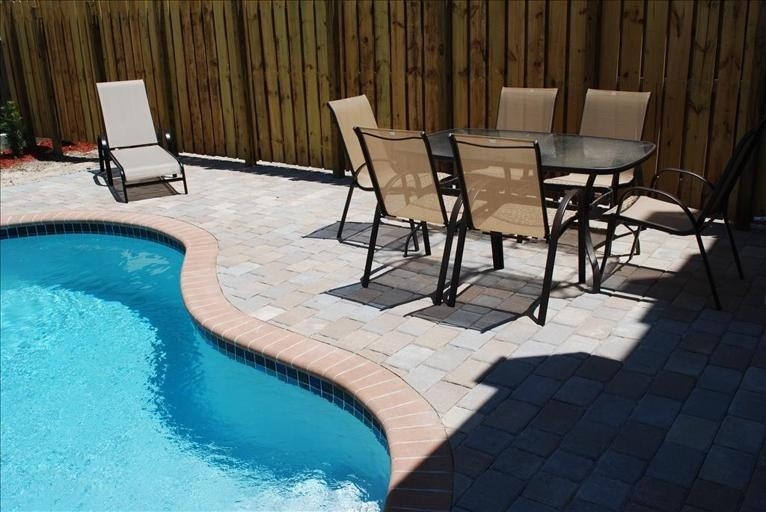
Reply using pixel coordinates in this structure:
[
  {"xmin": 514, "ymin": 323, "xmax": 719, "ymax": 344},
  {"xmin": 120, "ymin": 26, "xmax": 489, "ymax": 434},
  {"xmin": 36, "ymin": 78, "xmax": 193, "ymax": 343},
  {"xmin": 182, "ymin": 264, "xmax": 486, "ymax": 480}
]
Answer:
[
  {"xmin": 600, "ymin": 131, "xmax": 760, "ymax": 309},
  {"xmin": 544, "ymin": 90, "xmax": 650, "ymax": 253},
  {"xmin": 328, "ymin": 94, "xmax": 452, "ymax": 250},
  {"xmin": 494, "ymin": 87, "xmax": 557, "ymax": 243},
  {"xmin": 446, "ymin": 131, "xmax": 589, "ymax": 326},
  {"xmin": 95, "ymin": 78, "xmax": 187, "ymax": 202},
  {"xmin": 354, "ymin": 127, "xmax": 495, "ymax": 305}
]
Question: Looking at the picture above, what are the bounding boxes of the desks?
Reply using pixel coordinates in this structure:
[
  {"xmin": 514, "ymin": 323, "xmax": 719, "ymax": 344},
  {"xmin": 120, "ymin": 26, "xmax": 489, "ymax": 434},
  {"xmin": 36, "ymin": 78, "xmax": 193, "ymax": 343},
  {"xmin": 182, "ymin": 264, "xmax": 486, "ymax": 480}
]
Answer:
[{"xmin": 393, "ymin": 130, "xmax": 655, "ymax": 290}]
